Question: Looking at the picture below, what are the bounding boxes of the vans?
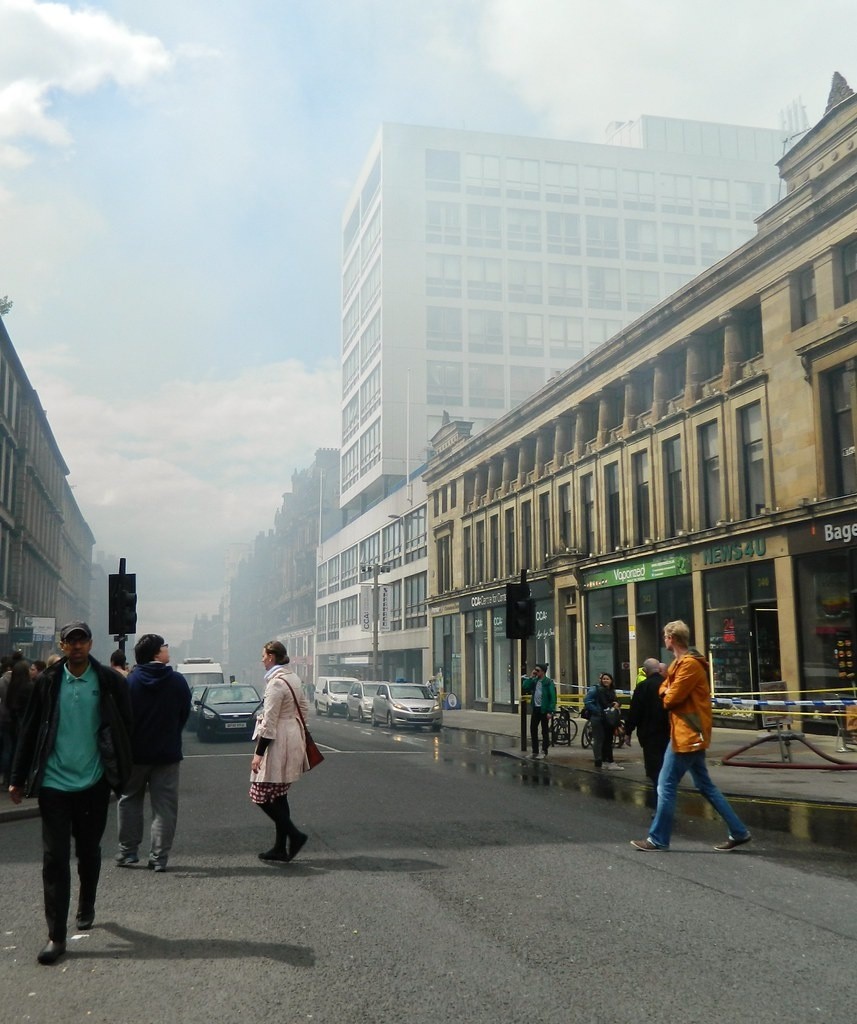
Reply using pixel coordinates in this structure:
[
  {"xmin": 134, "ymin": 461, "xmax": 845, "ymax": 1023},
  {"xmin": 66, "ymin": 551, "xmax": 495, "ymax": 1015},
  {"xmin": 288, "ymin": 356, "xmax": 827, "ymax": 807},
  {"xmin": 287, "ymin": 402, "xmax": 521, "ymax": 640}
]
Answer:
[
  {"xmin": 312, "ymin": 676, "xmax": 359, "ymax": 718},
  {"xmin": 175, "ymin": 658, "xmax": 236, "ymax": 718}
]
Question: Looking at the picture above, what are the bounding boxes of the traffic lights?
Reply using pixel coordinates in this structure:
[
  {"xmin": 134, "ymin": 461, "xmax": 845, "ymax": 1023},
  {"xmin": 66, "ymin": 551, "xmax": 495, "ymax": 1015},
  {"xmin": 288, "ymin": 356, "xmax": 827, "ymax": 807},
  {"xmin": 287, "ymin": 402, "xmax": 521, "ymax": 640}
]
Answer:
[
  {"xmin": 515, "ymin": 597, "xmax": 536, "ymax": 635},
  {"xmin": 115, "ymin": 589, "xmax": 137, "ymax": 627}
]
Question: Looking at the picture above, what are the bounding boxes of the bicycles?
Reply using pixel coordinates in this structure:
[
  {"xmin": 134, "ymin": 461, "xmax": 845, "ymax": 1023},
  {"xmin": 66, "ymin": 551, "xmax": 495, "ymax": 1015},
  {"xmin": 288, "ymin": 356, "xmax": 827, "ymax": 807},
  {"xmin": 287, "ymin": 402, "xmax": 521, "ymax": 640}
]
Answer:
[{"xmin": 549, "ymin": 704, "xmax": 627, "ymax": 749}]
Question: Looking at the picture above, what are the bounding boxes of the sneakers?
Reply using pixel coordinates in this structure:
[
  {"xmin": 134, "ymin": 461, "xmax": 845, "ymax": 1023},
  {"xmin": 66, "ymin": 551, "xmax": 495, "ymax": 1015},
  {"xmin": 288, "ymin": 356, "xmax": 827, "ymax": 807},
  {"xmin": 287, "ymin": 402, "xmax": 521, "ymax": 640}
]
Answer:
[
  {"xmin": 608, "ymin": 762, "xmax": 625, "ymax": 770},
  {"xmin": 714, "ymin": 831, "xmax": 753, "ymax": 851},
  {"xmin": 630, "ymin": 839, "xmax": 669, "ymax": 852},
  {"xmin": 601, "ymin": 762, "xmax": 609, "ymax": 769}
]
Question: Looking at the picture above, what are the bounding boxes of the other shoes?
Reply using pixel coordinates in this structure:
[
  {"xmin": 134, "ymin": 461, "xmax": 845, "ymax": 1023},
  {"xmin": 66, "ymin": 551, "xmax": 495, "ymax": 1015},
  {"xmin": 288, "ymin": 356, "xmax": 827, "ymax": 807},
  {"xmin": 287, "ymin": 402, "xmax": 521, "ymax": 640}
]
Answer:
[
  {"xmin": 148, "ymin": 858, "xmax": 168, "ymax": 871},
  {"xmin": 76, "ymin": 907, "xmax": 95, "ymax": 930},
  {"xmin": 258, "ymin": 849, "xmax": 288, "ymax": 863},
  {"xmin": 526, "ymin": 753, "xmax": 538, "ymax": 759},
  {"xmin": 114, "ymin": 853, "xmax": 139, "ymax": 864},
  {"xmin": 288, "ymin": 832, "xmax": 308, "ymax": 861},
  {"xmin": 38, "ymin": 940, "xmax": 66, "ymax": 963},
  {"xmin": 536, "ymin": 751, "xmax": 546, "ymax": 759}
]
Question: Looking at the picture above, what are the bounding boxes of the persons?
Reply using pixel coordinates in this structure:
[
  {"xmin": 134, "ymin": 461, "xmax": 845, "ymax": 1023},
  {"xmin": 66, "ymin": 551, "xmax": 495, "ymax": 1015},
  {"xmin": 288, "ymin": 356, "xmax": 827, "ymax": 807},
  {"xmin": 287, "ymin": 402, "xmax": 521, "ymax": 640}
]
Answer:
[
  {"xmin": 521, "ymin": 663, "xmax": 557, "ymax": 760},
  {"xmin": 632, "ymin": 620, "xmax": 752, "ymax": 852},
  {"xmin": 583, "ymin": 673, "xmax": 624, "ymax": 770},
  {"xmin": 249, "ymin": 641, "xmax": 308, "ymax": 862},
  {"xmin": 625, "ymin": 657, "xmax": 668, "ymax": 784},
  {"xmin": 307, "ymin": 681, "xmax": 315, "ymax": 702},
  {"xmin": 111, "ymin": 649, "xmax": 129, "ymax": 678},
  {"xmin": 114, "ymin": 634, "xmax": 192, "ymax": 871},
  {"xmin": 8, "ymin": 620, "xmax": 134, "ymax": 967},
  {"xmin": 0, "ymin": 652, "xmax": 53, "ymax": 791}
]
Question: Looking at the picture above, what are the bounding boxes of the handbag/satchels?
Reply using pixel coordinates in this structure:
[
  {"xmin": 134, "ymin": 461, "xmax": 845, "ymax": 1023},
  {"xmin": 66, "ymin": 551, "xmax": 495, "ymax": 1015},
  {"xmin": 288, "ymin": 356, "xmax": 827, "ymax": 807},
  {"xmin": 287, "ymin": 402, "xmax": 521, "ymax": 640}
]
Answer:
[
  {"xmin": 581, "ymin": 709, "xmax": 591, "ymax": 720},
  {"xmin": 602, "ymin": 708, "xmax": 619, "ymax": 729},
  {"xmin": 305, "ymin": 729, "xmax": 324, "ymax": 771},
  {"xmin": 641, "ymin": 718, "xmax": 665, "ymax": 752}
]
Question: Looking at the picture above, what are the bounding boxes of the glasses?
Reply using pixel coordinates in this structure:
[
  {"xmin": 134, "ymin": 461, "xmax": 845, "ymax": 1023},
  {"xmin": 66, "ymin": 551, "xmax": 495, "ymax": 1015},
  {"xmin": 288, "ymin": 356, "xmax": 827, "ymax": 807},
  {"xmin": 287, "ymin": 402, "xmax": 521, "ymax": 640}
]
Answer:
[
  {"xmin": 535, "ymin": 669, "xmax": 542, "ymax": 672},
  {"xmin": 62, "ymin": 636, "xmax": 90, "ymax": 645},
  {"xmin": 161, "ymin": 643, "xmax": 169, "ymax": 648}
]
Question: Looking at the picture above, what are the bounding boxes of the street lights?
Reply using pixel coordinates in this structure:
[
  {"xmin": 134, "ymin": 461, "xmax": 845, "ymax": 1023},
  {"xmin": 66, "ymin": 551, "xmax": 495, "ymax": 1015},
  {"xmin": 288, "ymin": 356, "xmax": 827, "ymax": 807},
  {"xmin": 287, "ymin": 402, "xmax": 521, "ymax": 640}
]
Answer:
[{"xmin": 358, "ymin": 560, "xmax": 394, "ymax": 682}]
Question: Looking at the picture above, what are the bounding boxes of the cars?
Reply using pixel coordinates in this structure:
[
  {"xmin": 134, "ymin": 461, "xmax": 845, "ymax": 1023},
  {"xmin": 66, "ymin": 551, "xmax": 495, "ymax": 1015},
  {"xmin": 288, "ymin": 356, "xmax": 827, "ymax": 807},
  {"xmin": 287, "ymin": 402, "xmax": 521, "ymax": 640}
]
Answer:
[
  {"xmin": 370, "ymin": 682, "xmax": 443, "ymax": 732},
  {"xmin": 195, "ymin": 683, "xmax": 265, "ymax": 742},
  {"xmin": 344, "ymin": 680, "xmax": 391, "ymax": 723}
]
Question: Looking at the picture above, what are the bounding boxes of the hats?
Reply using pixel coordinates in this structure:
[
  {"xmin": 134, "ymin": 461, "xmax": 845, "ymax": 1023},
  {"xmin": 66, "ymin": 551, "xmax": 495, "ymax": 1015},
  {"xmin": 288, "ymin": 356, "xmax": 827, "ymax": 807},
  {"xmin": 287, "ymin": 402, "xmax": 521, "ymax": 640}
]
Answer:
[
  {"xmin": 536, "ymin": 663, "xmax": 549, "ymax": 673},
  {"xmin": 59, "ymin": 619, "xmax": 92, "ymax": 641}
]
